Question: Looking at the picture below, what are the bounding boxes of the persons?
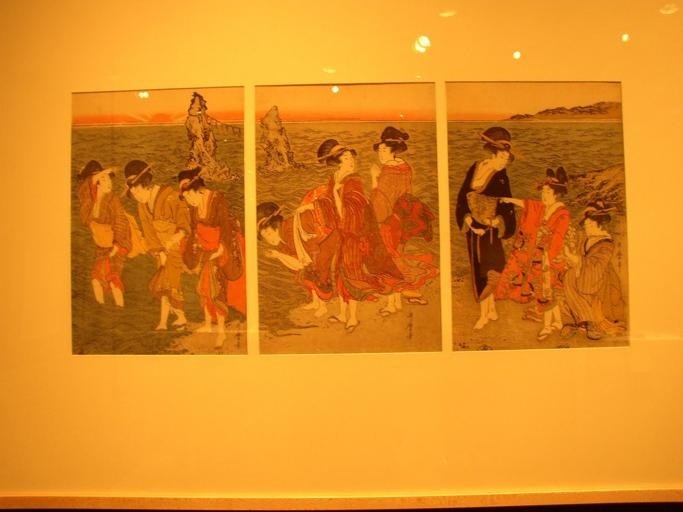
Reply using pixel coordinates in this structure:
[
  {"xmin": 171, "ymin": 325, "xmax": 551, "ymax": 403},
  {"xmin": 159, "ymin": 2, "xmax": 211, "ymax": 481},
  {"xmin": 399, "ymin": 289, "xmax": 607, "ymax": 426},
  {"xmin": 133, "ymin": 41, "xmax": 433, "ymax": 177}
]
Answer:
[
  {"xmin": 176, "ymin": 164, "xmax": 246, "ymax": 350},
  {"xmin": 456, "ymin": 126, "xmax": 628, "ymax": 344},
  {"xmin": 76, "ymin": 159, "xmax": 143, "ymax": 305},
  {"xmin": 118, "ymin": 160, "xmax": 195, "ymax": 332},
  {"xmin": 256, "ymin": 124, "xmax": 440, "ymax": 336}
]
